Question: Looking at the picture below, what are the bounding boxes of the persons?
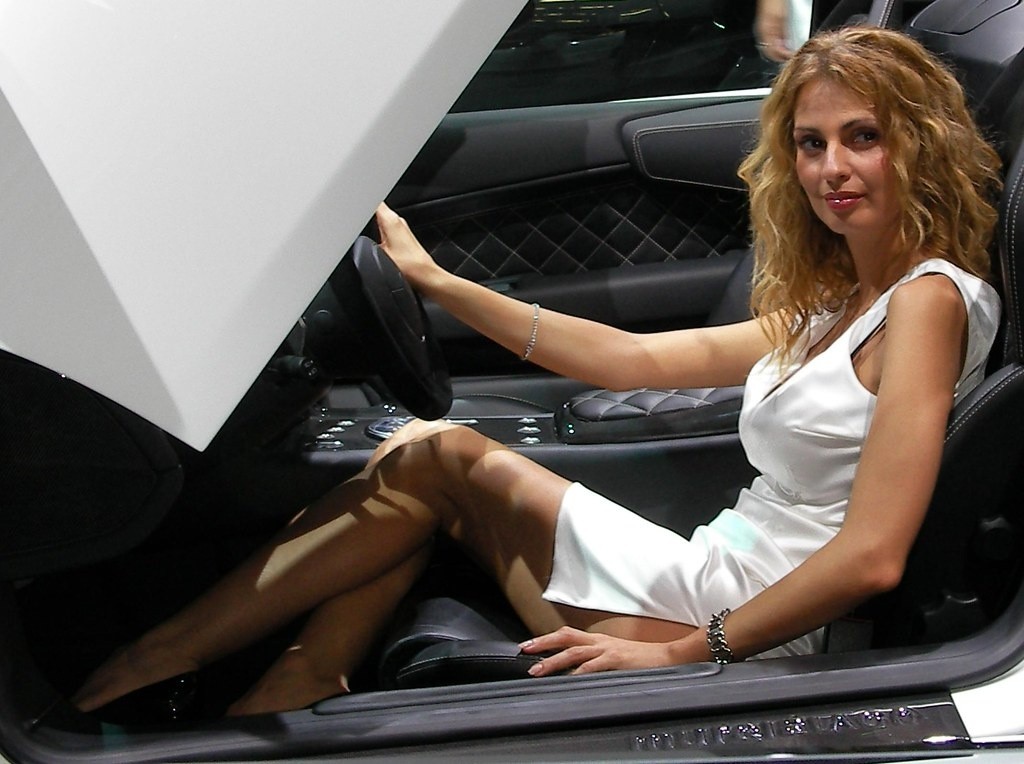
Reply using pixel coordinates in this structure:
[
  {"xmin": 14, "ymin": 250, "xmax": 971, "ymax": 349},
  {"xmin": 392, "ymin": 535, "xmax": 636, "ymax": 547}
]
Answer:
[{"xmin": 53, "ymin": 26, "xmax": 1001, "ymax": 732}]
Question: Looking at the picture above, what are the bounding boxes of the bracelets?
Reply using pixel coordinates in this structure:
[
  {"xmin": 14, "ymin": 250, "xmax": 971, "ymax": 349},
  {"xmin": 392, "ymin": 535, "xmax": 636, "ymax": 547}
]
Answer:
[
  {"xmin": 520, "ymin": 302, "xmax": 539, "ymax": 361},
  {"xmin": 706, "ymin": 607, "xmax": 736, "ymax": 665}
]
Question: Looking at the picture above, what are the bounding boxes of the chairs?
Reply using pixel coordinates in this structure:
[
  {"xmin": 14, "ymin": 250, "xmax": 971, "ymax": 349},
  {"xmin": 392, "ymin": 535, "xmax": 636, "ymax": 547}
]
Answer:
[
  {"xmin": 376, "ymin": 146, "xmax": 1024, "ymax": 691},
  {"xmin": 560, "ymin": 49, "xmax": 999, "ymax": 438}
]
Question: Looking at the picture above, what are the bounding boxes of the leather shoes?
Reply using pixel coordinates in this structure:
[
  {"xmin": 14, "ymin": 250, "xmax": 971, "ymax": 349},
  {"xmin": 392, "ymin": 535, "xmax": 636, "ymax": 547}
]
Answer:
[{"xmin": 52, "ymin": 671, "xmax": 206, "ymax": 731}]
